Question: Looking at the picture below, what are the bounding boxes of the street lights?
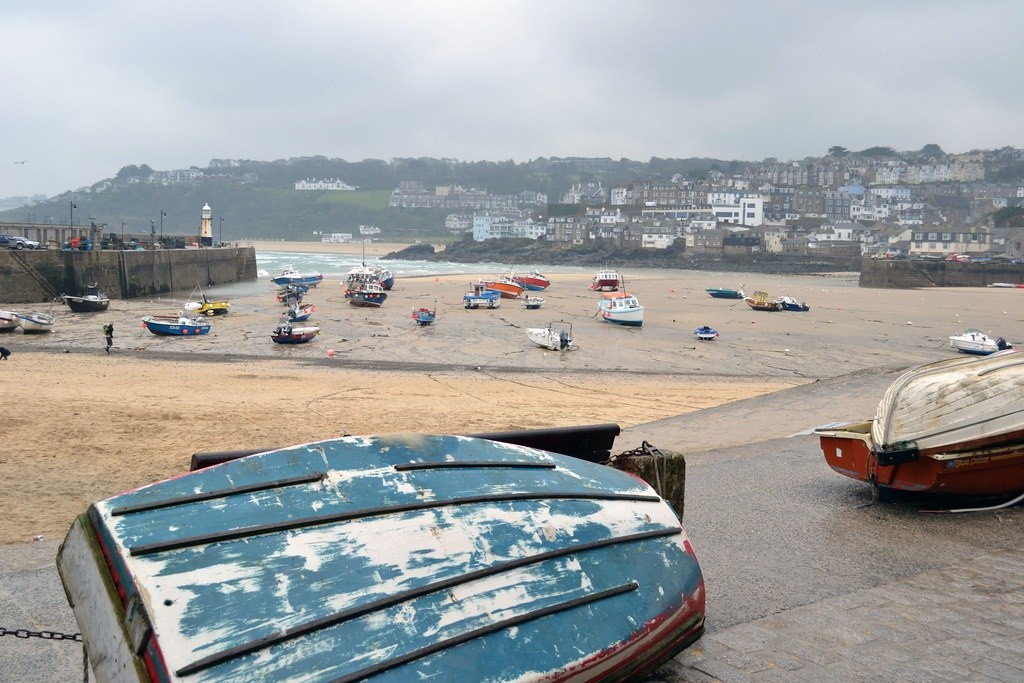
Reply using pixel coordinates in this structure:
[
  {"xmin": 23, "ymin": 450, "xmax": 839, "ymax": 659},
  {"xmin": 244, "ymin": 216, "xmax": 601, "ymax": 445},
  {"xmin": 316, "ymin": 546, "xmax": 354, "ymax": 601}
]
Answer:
[
  {"xmin": 219, "ymin": 215, "xmax": 225, "ymax": 243},
  {"xmin": 70, "ymin": 200, "xmax": 77, "ymax": 249},
  {"xmin": 88, "ymin": 217, "xmax": 95, "ymax": 238},
  {"xmin": 160, "ymin": 209, "xmax": 167, "ymax": 240}
]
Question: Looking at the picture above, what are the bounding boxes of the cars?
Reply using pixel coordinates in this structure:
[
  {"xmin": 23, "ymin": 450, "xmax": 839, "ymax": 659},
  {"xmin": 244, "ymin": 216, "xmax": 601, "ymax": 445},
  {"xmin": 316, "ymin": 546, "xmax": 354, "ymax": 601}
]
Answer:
[
  {"xmin": 0, "ymin": 234, "xmax": 41, "ymax": 251},
  {"xmin": 870, "ymin": 250, "xmax": 1024, "ymax": 264}
]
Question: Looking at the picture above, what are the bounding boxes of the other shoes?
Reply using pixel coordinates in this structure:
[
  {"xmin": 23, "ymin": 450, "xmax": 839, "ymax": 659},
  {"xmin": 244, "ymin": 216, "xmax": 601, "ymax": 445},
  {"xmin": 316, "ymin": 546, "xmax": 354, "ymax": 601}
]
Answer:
[{"xmin": 104, "ymin": 350, "xmax": 110, "ymax": 355}]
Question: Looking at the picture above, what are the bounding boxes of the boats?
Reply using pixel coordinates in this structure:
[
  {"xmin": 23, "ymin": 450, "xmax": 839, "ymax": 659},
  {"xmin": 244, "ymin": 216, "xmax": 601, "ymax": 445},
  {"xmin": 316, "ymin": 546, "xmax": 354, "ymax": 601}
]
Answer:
[
  {"xmin": 56, "ymin": 419, "xmax": 707, "ymax": 683},
  {"xmin": 464, "ymin": 285, "xmax": 501, "ymax": 309},
  {"xmin": 276, "ymin": 289, "xmax": 307, "ymax": 305},
  {"xmin": 948, "ymin": 330, "xmax": 1011, "ymax": 355},
  {"xmin": 409, "ymin": 307, "xmax": 436, "ymax": 324},
  {"xmin": 62, "ymin": 294, "xmax": 110, "ymax": 311},
  {"xmin": 142, "ymin": 313, "xmax": 211, "ymax": 335},
  {"xmin": 596, "ymin": 276, "xmax": 643, "ymax": 326},
  {"xmin": 521, "ymin": 295, "xmax": 544, "ymax": 308},
  {"xmin": 506, "ymin": 270, "xmax": 550, "ymax": 291},
  {"xmin": 0, "ymin": 299, "xmax": 55, "ymax": 333},
  {"xmin": 279, "ymin": 303, "xmax": 317, "ymax": 321},
  {"xmin": 184, "ymin": 279, "xmax": 230, "ymax": 315},
  {"xmin": 271, "ymin": 261, "xmax": 323, "ymax": 287},
  {"xmin": 696, "ymin": 326, "xmax": 719, "ymax": 341},
  {"xmin": 704, "ymin": 286, "xmax": 809, "ymax": 313},
  {"xmin": 590, "ymin": 264, "xmax": 619, "ymax": 292},
  {"xmin": 270, "ymin": 324, "xmax": 320, "ymax": 343},
  {"xmin": 344, "ymin": 243, "xmax": 394, "ymax": 307},
  {"xmin": 479, "ymin": 278, "xmax": 524, "ymax": 298},
  {"xmin": 813, "ymin": 350, "xmax": 1024, "ymax": 499},
  {"xmin": 525, "ymin": 321, "xmax": 573, "ymax": 351}
]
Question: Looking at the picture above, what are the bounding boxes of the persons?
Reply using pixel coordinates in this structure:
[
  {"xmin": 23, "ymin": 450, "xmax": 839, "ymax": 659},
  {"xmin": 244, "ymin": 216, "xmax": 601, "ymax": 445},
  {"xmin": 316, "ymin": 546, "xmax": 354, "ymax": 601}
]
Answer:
[
  {"xmin": 560, "ymin": 329, "xmax": 569, "ymax": 352},
  {"xmin": 0, "ymin": 347, "xmax": 11, "ymax": 360},
  {"xmin": 103, "ymin": 321, "xmax": 114, "ymax": 354}
]
